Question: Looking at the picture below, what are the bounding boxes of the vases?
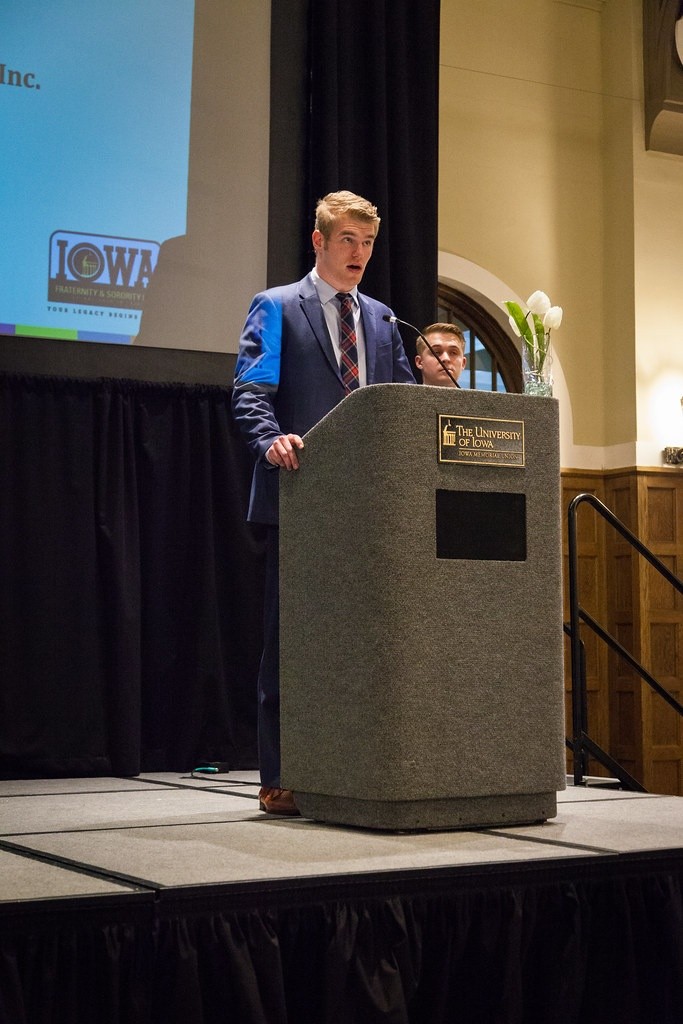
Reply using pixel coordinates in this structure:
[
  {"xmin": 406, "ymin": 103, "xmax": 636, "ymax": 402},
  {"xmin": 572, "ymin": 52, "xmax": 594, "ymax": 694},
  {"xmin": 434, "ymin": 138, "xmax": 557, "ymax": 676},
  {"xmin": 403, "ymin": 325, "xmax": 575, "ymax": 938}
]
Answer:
[{"xmin": 519, "ymin": 333, "xmax": 554, "ymax": 398}]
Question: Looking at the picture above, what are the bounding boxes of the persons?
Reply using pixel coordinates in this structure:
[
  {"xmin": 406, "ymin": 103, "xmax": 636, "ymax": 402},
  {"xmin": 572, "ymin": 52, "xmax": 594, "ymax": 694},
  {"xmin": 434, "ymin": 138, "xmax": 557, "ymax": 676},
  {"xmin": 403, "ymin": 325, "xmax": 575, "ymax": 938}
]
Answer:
[
  {"xmin": 232, "ymin": 189, "xmax": 422, "ymax": 814},
  {"xmin": 415, "ymin": 324, "xmax": 467, "ymax": 388}
]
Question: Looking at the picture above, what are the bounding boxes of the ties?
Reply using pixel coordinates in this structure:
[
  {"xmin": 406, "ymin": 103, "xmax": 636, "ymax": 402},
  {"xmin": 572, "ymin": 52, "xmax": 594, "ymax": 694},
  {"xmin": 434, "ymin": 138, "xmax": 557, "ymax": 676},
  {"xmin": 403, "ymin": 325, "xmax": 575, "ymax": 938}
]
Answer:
[{"xmin": 335, "ymin": 293, "xmax": 360, "ymax": 397}]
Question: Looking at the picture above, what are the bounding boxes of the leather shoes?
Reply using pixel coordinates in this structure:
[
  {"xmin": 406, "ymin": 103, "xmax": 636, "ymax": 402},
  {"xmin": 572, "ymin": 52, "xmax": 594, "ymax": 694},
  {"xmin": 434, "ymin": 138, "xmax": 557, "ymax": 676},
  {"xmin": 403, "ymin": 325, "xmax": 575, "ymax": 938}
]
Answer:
[{"xmin": 258, "ymin": 787, "xmax": 300, "ymax": 816}]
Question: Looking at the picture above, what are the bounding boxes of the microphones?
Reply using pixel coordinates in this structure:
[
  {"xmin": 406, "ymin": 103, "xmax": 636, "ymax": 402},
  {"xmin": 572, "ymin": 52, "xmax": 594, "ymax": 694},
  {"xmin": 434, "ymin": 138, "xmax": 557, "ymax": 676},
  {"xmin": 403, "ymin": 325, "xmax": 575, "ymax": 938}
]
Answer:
[{"xmin": 382, "ymin": 315, "xmax": 461, "ymax": 389}]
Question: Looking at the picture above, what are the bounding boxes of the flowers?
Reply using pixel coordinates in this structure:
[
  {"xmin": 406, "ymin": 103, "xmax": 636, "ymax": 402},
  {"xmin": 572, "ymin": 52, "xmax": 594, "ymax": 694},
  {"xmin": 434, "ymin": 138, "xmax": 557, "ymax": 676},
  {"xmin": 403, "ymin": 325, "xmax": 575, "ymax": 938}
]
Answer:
[{"xmin": 502, "ymin": 290, "xmax": 563, "ymax": 387}]
